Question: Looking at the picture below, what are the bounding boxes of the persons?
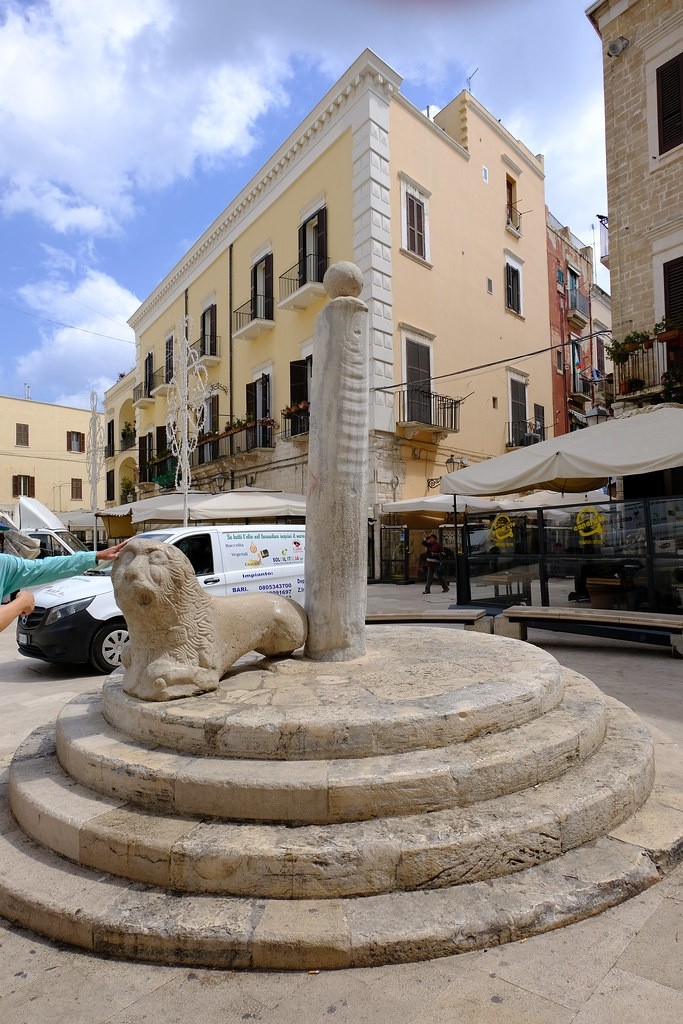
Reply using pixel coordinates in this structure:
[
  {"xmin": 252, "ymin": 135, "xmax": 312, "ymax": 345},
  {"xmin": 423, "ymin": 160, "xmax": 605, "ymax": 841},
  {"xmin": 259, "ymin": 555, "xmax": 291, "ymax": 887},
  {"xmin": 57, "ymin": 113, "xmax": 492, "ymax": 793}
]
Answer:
[
  {"xmin": 0, "ymin": 541, "xmax": 129, "ymax": 632},
  {"xmin": 422, "ymin": 534, "xmax": 451, "ymax": 594}
]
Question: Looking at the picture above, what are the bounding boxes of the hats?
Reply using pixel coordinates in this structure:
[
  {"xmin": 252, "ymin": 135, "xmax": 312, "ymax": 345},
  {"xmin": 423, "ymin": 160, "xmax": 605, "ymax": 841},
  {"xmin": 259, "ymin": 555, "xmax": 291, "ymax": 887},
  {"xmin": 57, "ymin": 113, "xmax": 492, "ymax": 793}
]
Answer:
[{"xmin": 0, "ymin": 521, "xmax": 10, "ymax": 530}]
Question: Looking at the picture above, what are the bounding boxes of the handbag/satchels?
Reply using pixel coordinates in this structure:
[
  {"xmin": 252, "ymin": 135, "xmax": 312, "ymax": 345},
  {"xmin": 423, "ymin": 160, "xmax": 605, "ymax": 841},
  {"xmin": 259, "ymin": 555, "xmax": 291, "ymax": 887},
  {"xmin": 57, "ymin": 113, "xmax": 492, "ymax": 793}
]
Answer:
[
  {"xmin": 489, "ymin": 560, "xmax": 494, "ymax": 570},
  {"xmin": 426, "ymin": 551, "xmax": 442, "ymax": 563}
]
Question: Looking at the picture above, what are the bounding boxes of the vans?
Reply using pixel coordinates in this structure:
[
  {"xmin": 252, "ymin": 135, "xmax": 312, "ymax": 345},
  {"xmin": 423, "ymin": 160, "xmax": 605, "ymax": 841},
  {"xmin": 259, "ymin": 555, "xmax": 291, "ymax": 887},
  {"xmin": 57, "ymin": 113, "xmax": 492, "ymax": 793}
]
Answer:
[{"xmin": 16, "ymin": 524, "xmax": 307, "ymax": 676}]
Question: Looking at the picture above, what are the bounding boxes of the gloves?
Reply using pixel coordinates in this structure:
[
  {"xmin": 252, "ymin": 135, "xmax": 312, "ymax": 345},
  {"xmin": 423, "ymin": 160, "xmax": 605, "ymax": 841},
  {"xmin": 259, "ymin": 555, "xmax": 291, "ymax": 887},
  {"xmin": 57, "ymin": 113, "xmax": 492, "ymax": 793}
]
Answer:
[{"xmin": 426, "ymin": 536, "xmax": 430, "ymax": 540}]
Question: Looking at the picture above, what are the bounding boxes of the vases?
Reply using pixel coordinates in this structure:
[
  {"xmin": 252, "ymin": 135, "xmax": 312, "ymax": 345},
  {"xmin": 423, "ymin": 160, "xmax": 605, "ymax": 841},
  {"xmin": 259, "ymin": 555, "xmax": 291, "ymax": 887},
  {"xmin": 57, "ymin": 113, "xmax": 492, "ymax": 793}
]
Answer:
[{"xmin": 619, "ymin": 382, "xmax": 632, "ymax": 395}]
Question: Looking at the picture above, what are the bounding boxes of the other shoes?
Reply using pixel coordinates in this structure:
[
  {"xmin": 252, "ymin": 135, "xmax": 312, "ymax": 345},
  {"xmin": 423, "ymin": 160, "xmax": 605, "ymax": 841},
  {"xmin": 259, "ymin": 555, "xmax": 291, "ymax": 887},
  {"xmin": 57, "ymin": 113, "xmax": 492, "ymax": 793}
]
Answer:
[
  {"xmin": 423, "ymin": 591, "xmax": 431, "ymax": 594},
  {"xmin": 442, "ymin": 587, "xmax": 450, "ymax": 592}
]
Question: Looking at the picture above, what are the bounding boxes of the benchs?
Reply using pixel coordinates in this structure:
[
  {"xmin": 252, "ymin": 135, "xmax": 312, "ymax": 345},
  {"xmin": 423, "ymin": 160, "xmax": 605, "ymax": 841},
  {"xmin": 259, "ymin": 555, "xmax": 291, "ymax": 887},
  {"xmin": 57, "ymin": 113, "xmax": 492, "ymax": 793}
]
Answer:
[
  {"xmin": 493, "ymin": 605, "xmax": 683, "ymax": 657},
  {"xmin": 364, "ymin": 608, "xmax": 486, "ymax": 634}
]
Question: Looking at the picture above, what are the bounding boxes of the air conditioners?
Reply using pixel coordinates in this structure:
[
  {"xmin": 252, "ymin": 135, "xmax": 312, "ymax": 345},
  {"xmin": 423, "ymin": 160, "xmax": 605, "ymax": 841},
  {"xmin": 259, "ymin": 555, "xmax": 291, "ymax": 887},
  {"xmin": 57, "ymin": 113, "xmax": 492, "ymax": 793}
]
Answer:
[{"xmin": 427, "ymin": 453, "xmax": 460, "ymax": 492}]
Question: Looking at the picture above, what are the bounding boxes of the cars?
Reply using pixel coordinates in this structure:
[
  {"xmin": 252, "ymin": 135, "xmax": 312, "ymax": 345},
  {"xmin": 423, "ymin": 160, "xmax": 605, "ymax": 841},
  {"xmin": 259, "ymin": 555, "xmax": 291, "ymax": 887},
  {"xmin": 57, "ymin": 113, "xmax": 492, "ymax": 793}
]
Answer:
[{"xmin": 466, "ymin": 528, "xmax": 516, "ymax": 574}]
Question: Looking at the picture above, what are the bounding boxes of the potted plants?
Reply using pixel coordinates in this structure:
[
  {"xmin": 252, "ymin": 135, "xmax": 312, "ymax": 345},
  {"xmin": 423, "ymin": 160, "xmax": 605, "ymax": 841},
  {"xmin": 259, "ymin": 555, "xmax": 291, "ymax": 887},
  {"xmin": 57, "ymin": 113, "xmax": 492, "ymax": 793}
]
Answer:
[
  {"xmin": 149, "ymin": 411, "xmax": 258, "ymax": 464},
  {"xmin": 283, "ymin": 399, "xmax": 309, "ymax": 415},
  {"xmin": 604, "ymin": 316, "xmax": 682, "ymax": 362}
]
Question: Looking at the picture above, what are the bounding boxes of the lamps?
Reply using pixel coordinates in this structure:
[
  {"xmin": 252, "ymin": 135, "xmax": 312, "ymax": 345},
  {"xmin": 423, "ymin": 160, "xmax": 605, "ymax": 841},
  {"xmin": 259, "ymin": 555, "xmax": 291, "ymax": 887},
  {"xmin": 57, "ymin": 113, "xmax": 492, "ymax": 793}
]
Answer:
[
  {"xmin": 606, "ymin": 36, "xmax": 630, "ymax": 57},
  {"xmin": 215, "ymin": 471, "xmax": 253, "ymax": 491}
]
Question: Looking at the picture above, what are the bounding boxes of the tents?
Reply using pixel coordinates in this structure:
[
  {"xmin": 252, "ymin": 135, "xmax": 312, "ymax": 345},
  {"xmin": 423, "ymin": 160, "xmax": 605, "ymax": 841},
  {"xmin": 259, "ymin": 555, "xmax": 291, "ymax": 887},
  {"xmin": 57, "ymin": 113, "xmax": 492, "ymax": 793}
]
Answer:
[
  {"xmin": 439, "ymin": 399, "xmax": 683, "ymax": 608},
  {"xmin": 93, "ymin": 486, "xmax": 308, "ymax": 552}
]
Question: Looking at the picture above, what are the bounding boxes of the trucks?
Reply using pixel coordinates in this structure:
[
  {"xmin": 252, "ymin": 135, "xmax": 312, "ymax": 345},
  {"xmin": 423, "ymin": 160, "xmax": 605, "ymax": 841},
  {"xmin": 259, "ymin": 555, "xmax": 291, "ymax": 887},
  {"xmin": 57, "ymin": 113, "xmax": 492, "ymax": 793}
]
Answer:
[{"xmin": 0, "ymin": 495, "xmax": 99, "ymax": 605}]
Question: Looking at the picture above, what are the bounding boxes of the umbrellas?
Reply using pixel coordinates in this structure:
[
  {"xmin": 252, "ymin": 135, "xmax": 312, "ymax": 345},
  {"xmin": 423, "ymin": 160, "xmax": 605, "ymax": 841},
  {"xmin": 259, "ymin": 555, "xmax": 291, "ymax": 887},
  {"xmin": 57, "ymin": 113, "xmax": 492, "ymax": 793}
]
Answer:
[{"xmin": 381, "ymin": 488, "xmax": 618, "ymax": 553}]
